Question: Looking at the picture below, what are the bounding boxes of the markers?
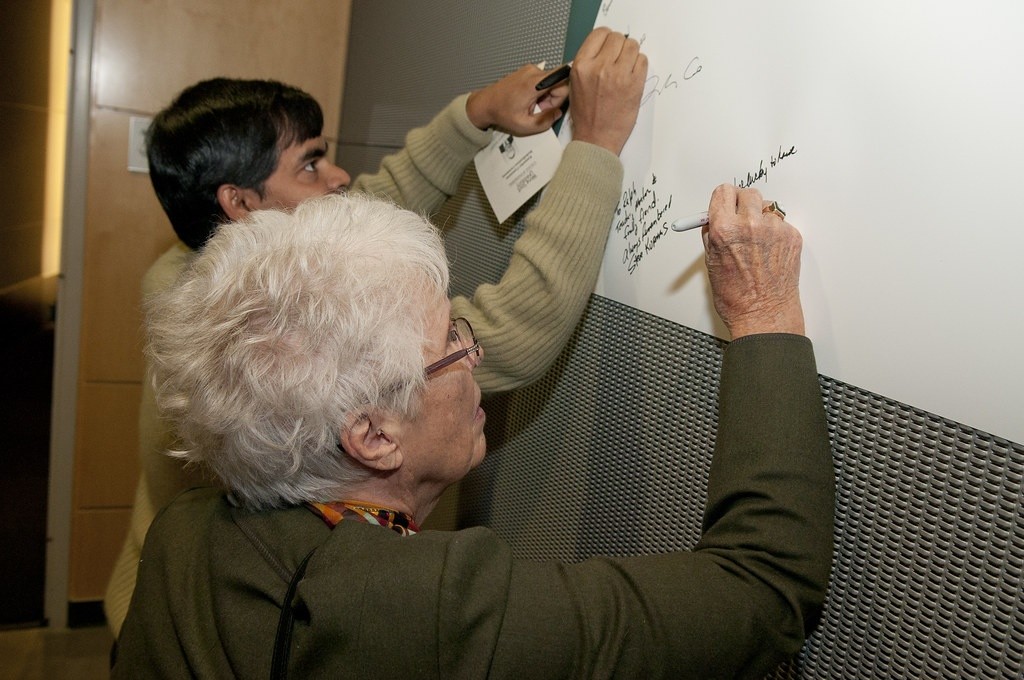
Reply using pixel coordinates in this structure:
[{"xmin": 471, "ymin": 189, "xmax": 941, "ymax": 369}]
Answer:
[
  {"xmin": 534, "ymin": 34, "xmax": 628, "ymax": 91},
  {"xmin": 671, "ymin": 211, "xmax": 709, "ymax": 232}
]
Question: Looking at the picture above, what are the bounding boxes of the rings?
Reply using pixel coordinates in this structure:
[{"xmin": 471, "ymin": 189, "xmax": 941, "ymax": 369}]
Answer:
[{"xmin": 762, "ymin": 202, "xmax": 786, "ymax": 220}]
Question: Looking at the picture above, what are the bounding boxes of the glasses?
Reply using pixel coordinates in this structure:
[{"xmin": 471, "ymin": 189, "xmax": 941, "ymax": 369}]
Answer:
[{"xmin": 348, "ymin": 315, "xmax": 484, "ymax": 408}]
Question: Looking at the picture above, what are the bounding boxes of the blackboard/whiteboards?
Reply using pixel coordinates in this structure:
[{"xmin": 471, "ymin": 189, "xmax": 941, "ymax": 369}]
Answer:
[{"xmin": 539, "ymin": 2, "xmax": 1024, "ymax": 447}]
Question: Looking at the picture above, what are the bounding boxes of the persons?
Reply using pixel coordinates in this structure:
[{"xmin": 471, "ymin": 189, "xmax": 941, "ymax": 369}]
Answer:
[
  {"xmin": 106, "ymin": 26, "xmax": 649, "ymax": 625},
  {"xmin": 110, "ymin": 184, "xmax": 836, "ymax": 680}
]
